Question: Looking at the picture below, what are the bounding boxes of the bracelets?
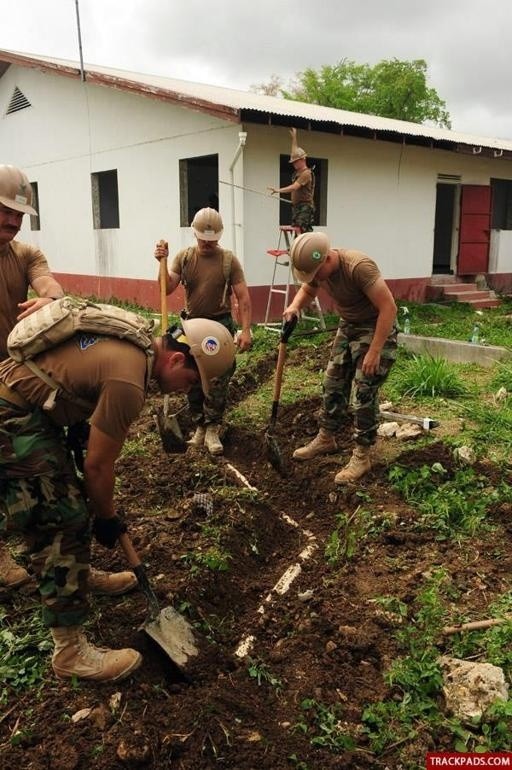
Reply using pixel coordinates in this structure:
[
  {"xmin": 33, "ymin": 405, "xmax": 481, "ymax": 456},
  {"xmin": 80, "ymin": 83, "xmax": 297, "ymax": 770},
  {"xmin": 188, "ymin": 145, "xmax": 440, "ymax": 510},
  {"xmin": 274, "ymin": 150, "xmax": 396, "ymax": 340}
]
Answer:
[{"xmin": 48, "ymin": 296, "xmax": 57, "ymax": 300}]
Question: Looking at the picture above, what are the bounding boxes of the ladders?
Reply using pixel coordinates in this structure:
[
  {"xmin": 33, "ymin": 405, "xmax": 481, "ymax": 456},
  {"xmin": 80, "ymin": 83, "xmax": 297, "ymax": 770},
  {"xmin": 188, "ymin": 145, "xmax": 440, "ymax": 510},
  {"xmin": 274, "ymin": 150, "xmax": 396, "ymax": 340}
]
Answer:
[{"xmin": 263, "ymin": 226, "xmax": 327, "ymax": 333}]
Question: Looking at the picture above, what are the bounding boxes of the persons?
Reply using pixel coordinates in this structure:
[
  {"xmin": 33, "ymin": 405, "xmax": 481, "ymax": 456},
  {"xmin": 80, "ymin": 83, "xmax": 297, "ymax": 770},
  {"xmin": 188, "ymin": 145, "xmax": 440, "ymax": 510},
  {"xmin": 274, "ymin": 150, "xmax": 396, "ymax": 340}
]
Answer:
[
  {"xmin": 281, "ymin": 232, "xmax": 399, "ymax": 486},
  {"xmin": 266, "ymin": 127, "xmax": 316, "ymax": 238},
  {"xmin": 1, "ymin": 167, "xmax": 67, "ymax": 588},
  {"xmin": 154, "ymin": 207, "xmax": 252, "ymax": 456},
  {"xmin": 1, "ymin": 315, "xmax": 236, "ymax": 684}
]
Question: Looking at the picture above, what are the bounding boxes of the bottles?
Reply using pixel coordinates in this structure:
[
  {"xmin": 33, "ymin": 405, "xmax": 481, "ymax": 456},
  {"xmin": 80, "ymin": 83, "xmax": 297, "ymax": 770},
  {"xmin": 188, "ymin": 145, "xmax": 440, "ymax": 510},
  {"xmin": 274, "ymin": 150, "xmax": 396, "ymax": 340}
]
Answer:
[
  {"xmin": 403, "ymin": 317, "xmax": 411, "ymax": 335},
  {"xmin": 471, "ymin": 325, "xmax": 480, "ymax": 345}
]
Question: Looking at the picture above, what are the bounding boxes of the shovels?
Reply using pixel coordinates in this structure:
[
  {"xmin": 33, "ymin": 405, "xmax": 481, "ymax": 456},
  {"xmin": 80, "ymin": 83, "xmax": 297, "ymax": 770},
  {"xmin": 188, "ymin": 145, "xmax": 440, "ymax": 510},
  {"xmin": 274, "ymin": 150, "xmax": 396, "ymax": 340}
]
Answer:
[
  {"xmin": 101, "ymin": 498, "xmax": 203, "ymax": 665},
  {"xmin": 265, "ymin": 323, "xmax": 289, "ymax": 475},
  {"xmin": 155, "ymin": 240, "xmax": 187, "ymax": 454}
]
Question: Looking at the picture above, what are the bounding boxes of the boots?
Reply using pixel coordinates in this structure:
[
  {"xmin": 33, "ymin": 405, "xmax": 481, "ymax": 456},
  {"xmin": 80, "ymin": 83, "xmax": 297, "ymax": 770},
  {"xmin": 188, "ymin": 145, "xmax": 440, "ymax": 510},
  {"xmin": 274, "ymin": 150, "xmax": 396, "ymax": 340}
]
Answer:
[
  {"xmin": 1, "ymin": 548, "xmax": 32, "ymax": 589},
  {"xmin": 334, "ymin": 442, "xmax": 372, "ymax": 484},
  {"xmin": 50, "ymin": 625, "xmax": 142, "ymax": 685},
  {"xmin": 186, "ymin": 426, "xmax": 207, "ymax": 447},
  {"xmin": 294, "ymin": 428, "xmax": 338, "ymax": 460},
  {"xmin": 88, "ymin": 564, "xmax": 140, "ymax": 598},
  {"xmin": 203, "ymin": 425, "xmax": 223, "ymax": 455}
]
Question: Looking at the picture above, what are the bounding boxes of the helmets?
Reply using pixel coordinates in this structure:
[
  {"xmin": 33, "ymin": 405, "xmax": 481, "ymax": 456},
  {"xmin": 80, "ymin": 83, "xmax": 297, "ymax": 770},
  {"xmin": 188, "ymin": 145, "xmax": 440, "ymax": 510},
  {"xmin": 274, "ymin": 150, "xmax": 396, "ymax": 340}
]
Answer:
[
  {"xmin": 289, "ymin": 147, "xmax": 306, "ymax": 163},
  {"xmin": 1, "ymin": 164, "xmax": 38, "ymax": 216},
  {"xmin": 179, "ymin": 316, "xmax": 236, "ymax": 395},
  {"xmin": 191, "ymin": 208, "xmax": 224, "ymax": 242},
  {"xmin": 289, "ymin": 233, "xmax": 330, "ymax": 285}
]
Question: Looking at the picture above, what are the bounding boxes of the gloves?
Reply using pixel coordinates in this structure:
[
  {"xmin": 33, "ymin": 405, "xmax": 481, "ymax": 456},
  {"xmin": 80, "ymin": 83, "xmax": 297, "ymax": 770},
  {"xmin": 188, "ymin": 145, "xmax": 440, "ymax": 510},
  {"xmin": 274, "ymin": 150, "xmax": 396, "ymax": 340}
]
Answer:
[{"xmin": 93, "ymin": 515, "xmax": 128, "ymax": 549}]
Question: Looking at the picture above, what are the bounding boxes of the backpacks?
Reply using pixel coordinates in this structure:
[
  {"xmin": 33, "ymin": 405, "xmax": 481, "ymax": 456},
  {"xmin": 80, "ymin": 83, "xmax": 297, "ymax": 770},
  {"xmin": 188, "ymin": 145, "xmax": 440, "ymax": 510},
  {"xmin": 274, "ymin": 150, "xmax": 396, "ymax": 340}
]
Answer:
[{"xmin": 8, "ymin": 295, "xmax": 155, "ymax": 412}]
